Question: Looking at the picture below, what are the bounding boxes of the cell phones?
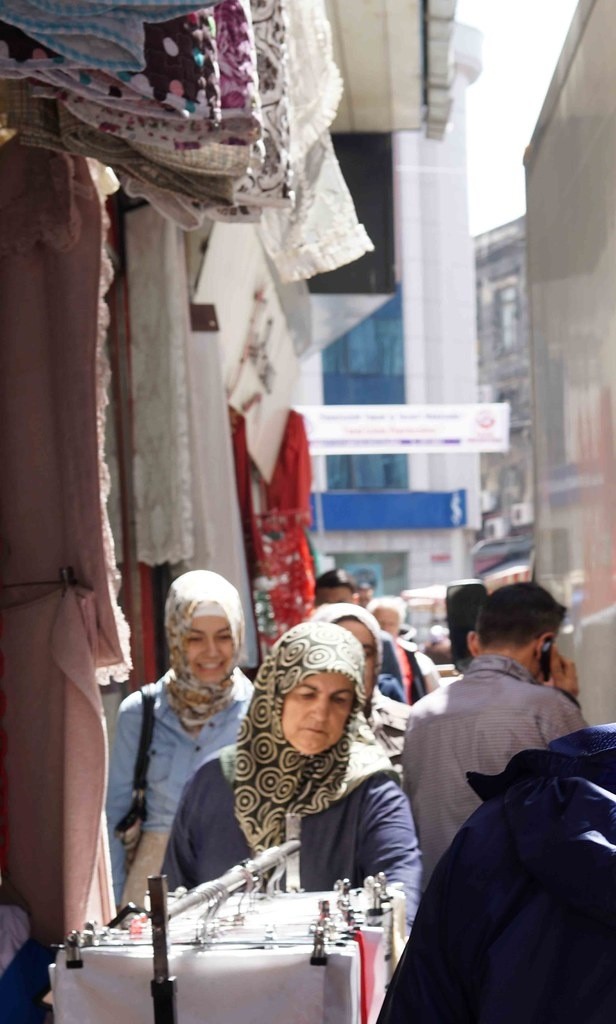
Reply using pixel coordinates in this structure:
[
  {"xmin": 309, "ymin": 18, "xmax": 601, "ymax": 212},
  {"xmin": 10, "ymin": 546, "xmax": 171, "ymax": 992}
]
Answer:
[{"xmin": 540, "ymin": 639, "xmax": 554, "ymax": 682}]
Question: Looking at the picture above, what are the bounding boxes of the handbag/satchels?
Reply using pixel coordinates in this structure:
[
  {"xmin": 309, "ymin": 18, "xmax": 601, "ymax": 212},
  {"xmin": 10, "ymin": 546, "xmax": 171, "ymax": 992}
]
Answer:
[{"xmin": 115, "ymin": 682, "xmax": 156, "ymax": 874}]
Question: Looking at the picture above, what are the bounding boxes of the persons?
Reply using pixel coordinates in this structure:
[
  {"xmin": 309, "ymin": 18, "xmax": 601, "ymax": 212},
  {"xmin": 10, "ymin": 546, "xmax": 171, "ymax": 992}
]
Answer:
[
  {"xmin": 104, "ymin": 569, "xmax": 256, "ymax": 907},
  {"xmin": 315, "ymin": 568, "xmax": 355, "ymax": 606},
  {"xmin": 302, "ymin": 603, "xmax": 411, "ymax": 786},
  {"xmin": 158, "ymin": 622, "xmax": 424, "ymax": 937},
  {"xmin": 365, "ymin": 596, "xmax": 441, "ymax": 705},
  {"xmin": 375, "ymin": 720, "xmax": 615, "ymax": 1024},
  {"xmin": 404, "ymin": 581, "xmax": 590, "ymax": 874},
  {"xmin": 0, "ymin": 874, "xmax": 54, "ymax": 1024}
]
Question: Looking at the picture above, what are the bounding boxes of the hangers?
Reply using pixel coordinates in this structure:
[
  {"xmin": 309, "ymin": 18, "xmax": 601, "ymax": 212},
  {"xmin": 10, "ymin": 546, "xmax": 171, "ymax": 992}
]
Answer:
[{"xmin": 50, "ymin": 845, "xmax": 393, "ymax": 968}]
